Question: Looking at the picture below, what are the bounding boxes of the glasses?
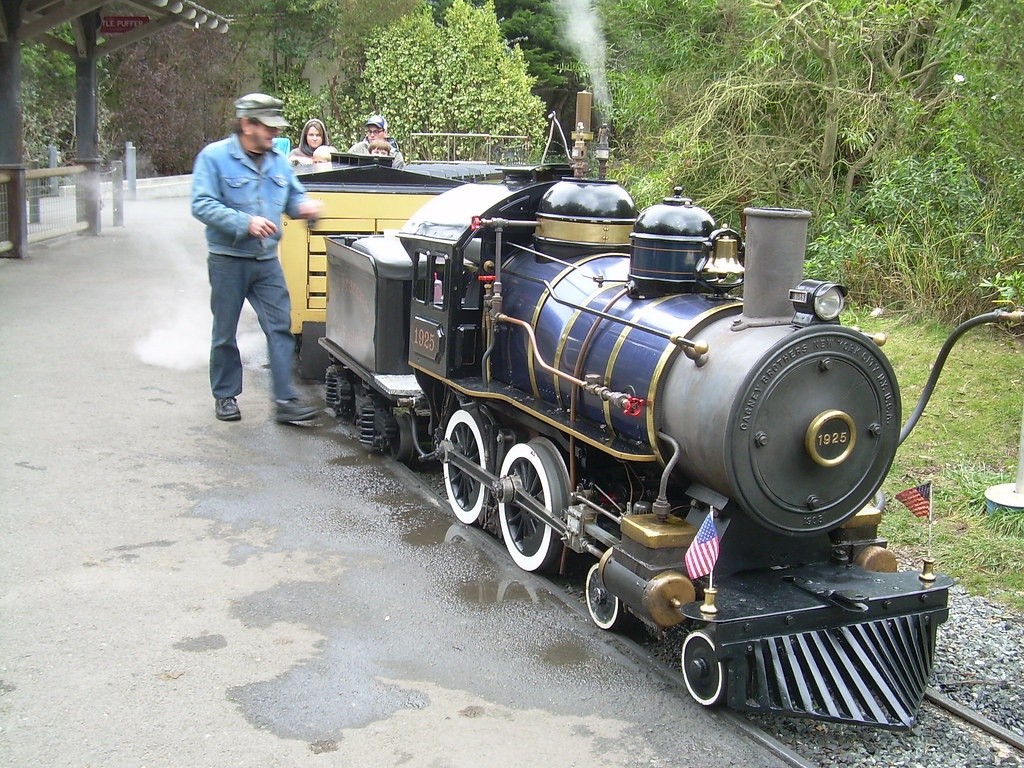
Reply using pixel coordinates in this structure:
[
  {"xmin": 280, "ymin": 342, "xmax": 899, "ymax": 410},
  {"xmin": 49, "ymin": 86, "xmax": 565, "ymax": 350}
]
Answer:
[{"xmin": 367, "ymin": 130, "xmax": 382, "ymax": 135}]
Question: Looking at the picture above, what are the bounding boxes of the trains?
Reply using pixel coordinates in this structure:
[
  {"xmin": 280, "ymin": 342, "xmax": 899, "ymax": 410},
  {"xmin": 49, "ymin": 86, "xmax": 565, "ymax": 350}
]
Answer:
[{"xmin": 268, "ymin": 88, "xmax": 1024, "ymax": 736}]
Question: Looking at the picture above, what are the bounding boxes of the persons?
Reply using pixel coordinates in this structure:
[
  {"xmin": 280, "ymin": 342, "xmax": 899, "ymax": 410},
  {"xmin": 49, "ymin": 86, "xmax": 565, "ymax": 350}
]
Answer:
[
  {"xmin": 347, "ymin": 114, "xmax": 404, "ymax": 174},
  {"xmin": 311, "ymin": 145, "xmax": 336, "ymax": 166},
  {"xmin": 367, "ymin": 139, "xmax": 391, "ymax": 160},
  {"xmin": 286, "ymin": 118, "xmax": 336, "ymax": 167},
  {"xmin": 189, "ymin": 92, "xmax": 324, "ymax": 422}
]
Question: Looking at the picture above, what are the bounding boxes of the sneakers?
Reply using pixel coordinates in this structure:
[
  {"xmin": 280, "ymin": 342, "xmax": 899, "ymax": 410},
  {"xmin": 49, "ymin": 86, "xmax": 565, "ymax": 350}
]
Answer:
[
  {"xmin": 276, "ymin": 400, "xmax": 320, "ymax": 422},
  {"xmin": 215, "ymin": 397, "xmax": 241, "ymax": 420}
]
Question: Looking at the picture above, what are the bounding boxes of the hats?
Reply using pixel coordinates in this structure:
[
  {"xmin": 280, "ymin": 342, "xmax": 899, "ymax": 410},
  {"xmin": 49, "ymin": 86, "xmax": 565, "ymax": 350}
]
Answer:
[
  {"xmin": 233, "ymin": 94, "xmax": 291, "ymax": 127},
  {"xmin": 365, "ymin": 115, "xmax": 388, "ymax": 132}
]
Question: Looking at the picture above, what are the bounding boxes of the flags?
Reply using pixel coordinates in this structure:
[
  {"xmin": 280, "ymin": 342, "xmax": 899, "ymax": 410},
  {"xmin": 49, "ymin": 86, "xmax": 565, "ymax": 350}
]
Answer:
[
  {"xmin": 895, "ymin": 481, "xmax": 929, "ymax": 517},
  {"xmin": 685, "ymin": 514, "xmax": 720, "ymax": 579}
]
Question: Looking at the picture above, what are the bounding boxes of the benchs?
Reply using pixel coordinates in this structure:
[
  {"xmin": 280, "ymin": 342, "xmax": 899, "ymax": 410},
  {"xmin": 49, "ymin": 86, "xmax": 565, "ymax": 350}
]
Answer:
[{"xmin": 351, "ymin": 236, "xmax": 443, "ymax": 283}]
